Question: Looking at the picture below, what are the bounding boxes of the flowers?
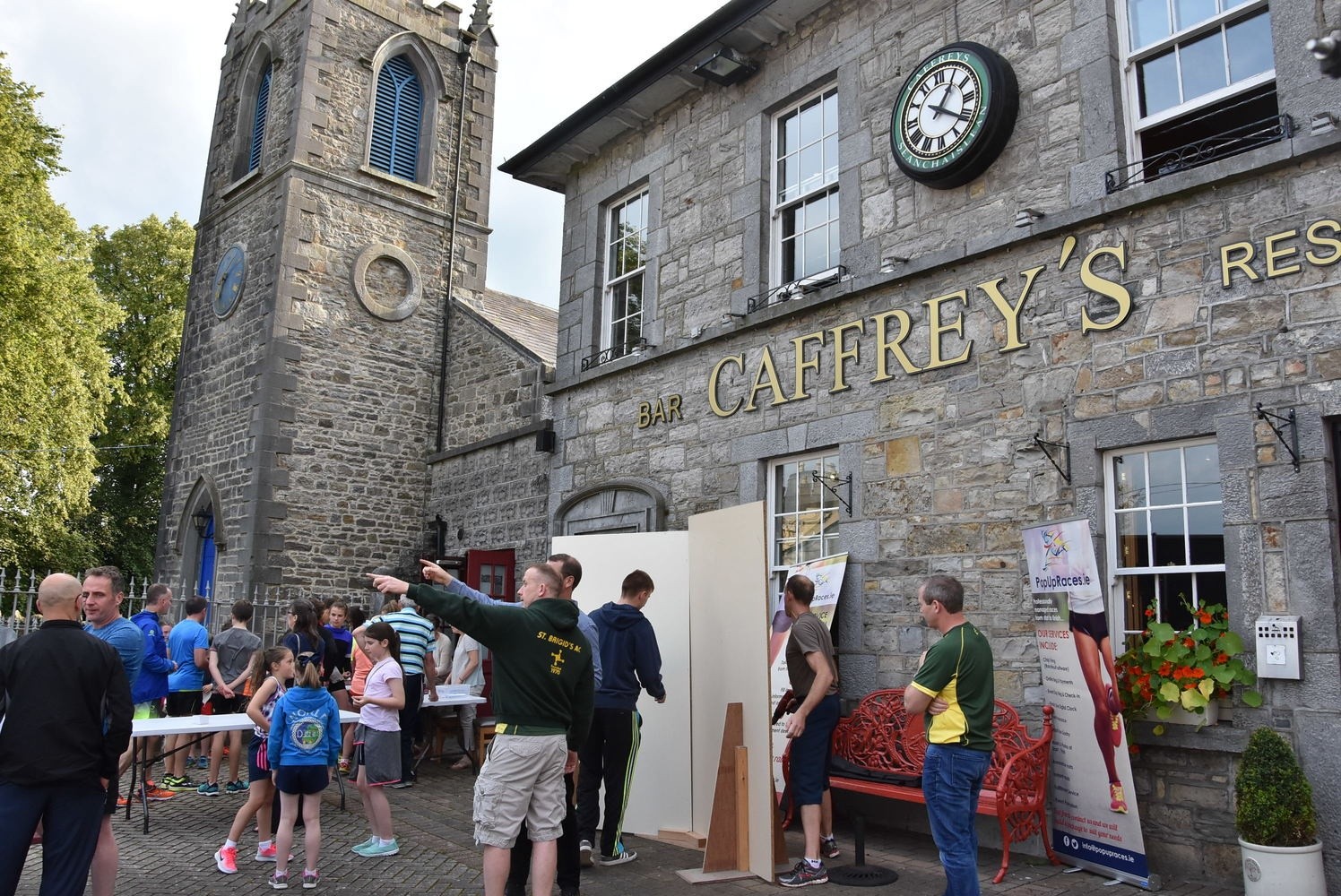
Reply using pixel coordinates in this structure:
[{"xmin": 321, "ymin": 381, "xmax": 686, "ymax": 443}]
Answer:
[{"xmin": 1114, "ymin": 591, "xmax": 1263, "ymax": 753}]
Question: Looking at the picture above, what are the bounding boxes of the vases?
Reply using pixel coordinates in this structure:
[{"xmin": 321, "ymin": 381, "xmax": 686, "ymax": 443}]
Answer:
[{"xmin": 1133, "ymin": 699, "xmax": 1219, "ymax": 723}]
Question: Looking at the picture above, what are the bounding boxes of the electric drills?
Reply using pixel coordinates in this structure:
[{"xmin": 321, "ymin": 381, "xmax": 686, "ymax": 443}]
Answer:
[{"xmin": 772, "ymin": 690, "xmax": 799, "ymax": 725}]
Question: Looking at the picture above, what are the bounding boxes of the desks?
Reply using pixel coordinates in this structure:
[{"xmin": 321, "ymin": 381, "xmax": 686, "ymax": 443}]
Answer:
[
  {"xmin": 419, "ymin": 693, "xmax": 488, "ymax": 775},
  {"xmin": 124, "ymin": 710, "xmax": 361, "ymax": 836}
]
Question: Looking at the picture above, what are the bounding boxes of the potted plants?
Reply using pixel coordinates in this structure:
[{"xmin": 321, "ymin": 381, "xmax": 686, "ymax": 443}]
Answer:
[{"xmin": 1233, "ymin": 725, "xmax": 1328, "ymax": 896}]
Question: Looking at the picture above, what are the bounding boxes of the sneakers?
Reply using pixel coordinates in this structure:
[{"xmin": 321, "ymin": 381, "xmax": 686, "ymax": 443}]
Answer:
[
  {"xmin": 817, "ymin": 836, "xmax": 840, "ymax": 858},
  {"xmin": 255, "ymin": 844, "xmax": 294, "ymax": 862},
  {"xmin": 106, "ymin": 787, "xmax": 129, "ymax": 807},
  {"xmin": 359, "ymin": 840, "xmax": 399, "ymax": 857},
  {"xmin": 600, "ymin": 849, "xmax": 637, "ymax": 865},
  {"xmin": 777, "ymin": 858, "xmax": 829, "ymax": 887},
  {"xmin": 184, "ymin": 756, "xmax": 197, "ymax": 768},
  {"xmin": 214, "ymin": 845, "xmax": 238, "ymax": 874},
  {"xmin": 160, "ymin": 773, "xmax": 174, "ymax": 789},
  {"xmin": 225, "ymin": 777, "xmax": 250, "ymax": 793},
  {"xmin": 352, "ymin": 837, "xmax": 379, "ymax": 854},
  {"xmin": 302, "ymin": 869, "xmax": 320, "ymax": 888},
  {"xmin": 197, "ymin": 780, "xmax": 219, "ymax": 796},
  {"xmin": 268, "ymin": 870, "xmax": 288, "ymax": 890},
  {"xmin": 578, "ymin": 840, "xmax": 594, "ymax": 866},
  {"xmin": 169, "ymin": 773, "xmax": 200, "ymax": 791},
  {"xmin": 198, "ymin": 755, "xmax": 209, "ymax": 768},
  {"xmin": 136, "ymin": 781, "xmax": 175, "ymax": 801}
]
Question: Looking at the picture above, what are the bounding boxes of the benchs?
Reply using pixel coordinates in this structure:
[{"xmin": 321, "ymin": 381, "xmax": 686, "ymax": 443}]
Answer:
[
  {"xmin": 783, "ymin": 689, "xmax": 1060, "ymax": 883},
  {"xmin": 436, "ymin": 713, "xmax": 496, "ymax": 768}
]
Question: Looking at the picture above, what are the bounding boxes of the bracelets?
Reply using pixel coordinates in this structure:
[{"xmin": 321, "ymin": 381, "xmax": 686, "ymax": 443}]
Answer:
[{"xmin": 459, "ymin": 677, "xmax": 464, "ymax": 682}]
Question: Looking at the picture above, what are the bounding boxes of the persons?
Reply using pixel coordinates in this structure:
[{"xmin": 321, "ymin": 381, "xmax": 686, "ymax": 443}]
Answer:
[
  {"xmin": 367, "ymin": 564, "xmax": 595, "ymax": 896},
  {"xmin": 904, "ymin": 575, "xmax": 996, "ymax": 895},
  {"xmin": 79, "ymin": 566, "xmax": 145, "ymax": 896},
  {"xmin": 1058, "ymin": 519, "xmax": 1130, "ymax": 815},
  {"xmin": 579, "ymin": 568, "xmax": 667, "ymax": 870},
  {"xmin": 420, "ymin": 553, "xmax": 602, "ymax": 896},
  {"xmin": 0, "ymin": 572, "xmax": 136, "ymax": 896},
  {"xmin": 776, "ymin": 575, "xmax": 841, "ymax": 888},
  {"xmin": 129, "ymin": 584, "xmax": 486, "ymax": 891}
]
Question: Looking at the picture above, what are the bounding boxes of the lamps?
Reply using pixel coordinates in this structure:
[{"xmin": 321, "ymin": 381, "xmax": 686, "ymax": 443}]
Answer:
[
  {"xmin": 878, "ymin": 255, "xmax": 908, "ymax": 274},
  {"xmin": 1014, "ymin": 208, "xmax": 1044, "ymax": 229},
  {"xmin": 535, "ymin": 429, "xmax": 555, "ymax": 455},
  {"xmin": 693, "ymin": 47, "xmax": 759, "ymax": 87},
  {"xmin": 427, "ymin": 515, "xmax": 447, "ymax": 530},
  {"xmin": 1309, "ymin": 111, "xmax": 1340, "ymax": 137},
  {"xmin": 191, "ymin": 505, "xmax": 214, "ymax": 540},
  {"xmin": 632, "ymin": 344, "xmax": 656, "ymax": 356},
  {"xmin": 722, "ymin": 313, "xmax": 746, "ymax": 326}
]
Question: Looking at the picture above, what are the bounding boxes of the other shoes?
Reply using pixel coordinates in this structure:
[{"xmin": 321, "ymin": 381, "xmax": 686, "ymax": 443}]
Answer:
[
  {"xmin": 383, "ymin": 778, "xmax": 412, "ymax": 788},
  {"xmin": 208, "ymin": 745, "xmax": 230, "ymax": 759},
  {"xmin": 451, "ymin": 755, "xmax": 472, "ymax": 769},
  {"xmin": 338, "ymin": 759, "xmax": 353, "ymax": 774}
]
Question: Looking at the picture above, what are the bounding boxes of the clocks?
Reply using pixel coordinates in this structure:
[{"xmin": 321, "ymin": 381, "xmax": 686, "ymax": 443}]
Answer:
[
  {"xmin": 212, "ymin": 248, "xmax": 243, "ymax": 313},
  {"xmin": 905, "ymin": 67, "xmax": 975, "ymax": 153}
]
